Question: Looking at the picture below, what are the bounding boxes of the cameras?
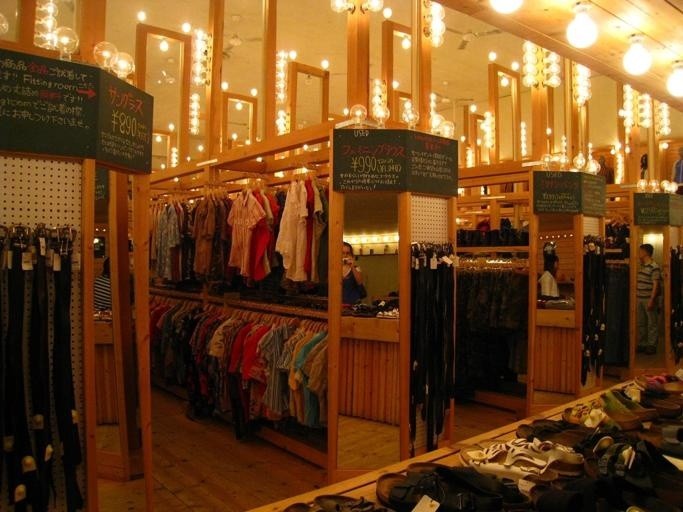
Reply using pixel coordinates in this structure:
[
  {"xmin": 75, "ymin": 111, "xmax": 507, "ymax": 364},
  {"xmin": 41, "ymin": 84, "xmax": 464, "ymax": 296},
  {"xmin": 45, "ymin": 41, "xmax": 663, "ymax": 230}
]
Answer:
[{"xmin": 343, "ymin": 258, "xmax": 349, "ymax": 264}]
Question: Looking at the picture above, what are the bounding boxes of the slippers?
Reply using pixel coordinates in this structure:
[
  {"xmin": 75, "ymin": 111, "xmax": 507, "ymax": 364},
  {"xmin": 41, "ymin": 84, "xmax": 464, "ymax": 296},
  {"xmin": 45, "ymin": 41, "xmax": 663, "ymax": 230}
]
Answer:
[{"xmin": 285, "ymin": 371, "xmax": 683, "ymax": 512}]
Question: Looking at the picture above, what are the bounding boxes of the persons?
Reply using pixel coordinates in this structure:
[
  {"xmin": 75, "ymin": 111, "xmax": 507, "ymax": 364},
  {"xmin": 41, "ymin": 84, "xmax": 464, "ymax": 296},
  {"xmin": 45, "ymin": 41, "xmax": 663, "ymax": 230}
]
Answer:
[
  {"xmin": 637, "ymin": 154, "xmax": 649, "ymax": 182},
  {"xmin": 536, "ymin": 254, "xmax": 561, "ymax": 300},
  {"xmin": 340, "ymin": 241, "xmax": 368, "ymax": 305},
  {"xmin": 594, "ymin": 156, "xmax": 615, "ymax": 184},
  {"xmin": 635, "ymin": 243, "xmax": 662, "ymax": 355},
  {"xmin": 670, "ymin": 147, "xmax": 683, "ymax": 185},
  {"xmin": 473, "ymin": 220, "xmax": 489, "ymax": 239},
  {"xmin": 93, "ymin": 256, "xmax": 111, "ymax": 313}
]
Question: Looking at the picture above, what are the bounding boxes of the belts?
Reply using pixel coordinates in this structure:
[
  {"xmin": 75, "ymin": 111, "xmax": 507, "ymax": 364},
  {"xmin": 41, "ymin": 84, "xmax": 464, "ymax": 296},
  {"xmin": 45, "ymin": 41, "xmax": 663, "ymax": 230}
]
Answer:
[
  {"xmin": 582, "ymin": 235, "xmax": 604, "ymax": 386},
  {"xmin": 409, "ymin": 242, "xmax": 453, "ymax": 452},
  {"xmin": 669, "ymin": 246, "xmax": 682, "ymax": 364},
  {"xmin": 2, "ymin": 224, "xmax": 86, "ymax": 512}
]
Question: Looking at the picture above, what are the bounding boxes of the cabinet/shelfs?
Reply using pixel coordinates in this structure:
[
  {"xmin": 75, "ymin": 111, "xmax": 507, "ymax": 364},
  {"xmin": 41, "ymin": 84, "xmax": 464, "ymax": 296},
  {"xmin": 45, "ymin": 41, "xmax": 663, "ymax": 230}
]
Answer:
[{"xmin": 0, "ymin": 39, "xmax": 683, "ymax": 512}]
